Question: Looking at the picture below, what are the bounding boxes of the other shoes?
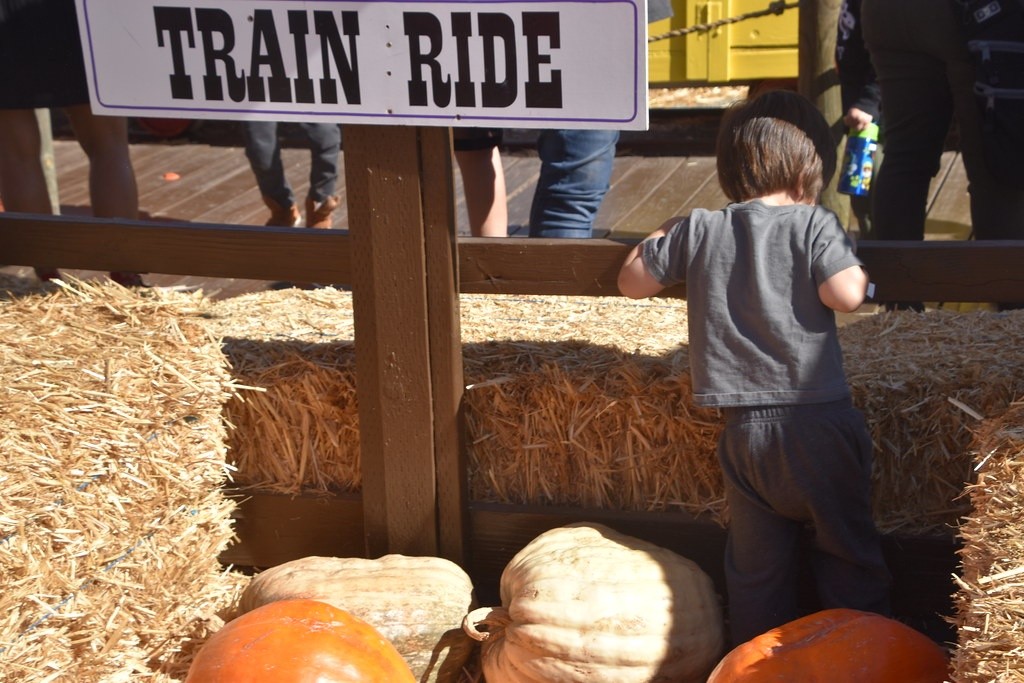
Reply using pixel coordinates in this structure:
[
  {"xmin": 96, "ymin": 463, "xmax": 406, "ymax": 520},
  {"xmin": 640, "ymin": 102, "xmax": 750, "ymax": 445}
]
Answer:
[
  {"xmin": 306, "ymin": 194, "xmax": 338, "ymax": 230},
  {"xmin": 264, "ymin": 206, "xmax": 301, "ymax": 227},
  {"xmin": 110, "ymin": 273, "xmax": 148, "ymax": 295}
]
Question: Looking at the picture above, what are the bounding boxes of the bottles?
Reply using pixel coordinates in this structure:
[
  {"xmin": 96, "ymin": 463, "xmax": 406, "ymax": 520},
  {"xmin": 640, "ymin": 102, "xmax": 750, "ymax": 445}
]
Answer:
[{"xmin": 837, "ymin": 122, "xmax": 879, "ymax": 196}]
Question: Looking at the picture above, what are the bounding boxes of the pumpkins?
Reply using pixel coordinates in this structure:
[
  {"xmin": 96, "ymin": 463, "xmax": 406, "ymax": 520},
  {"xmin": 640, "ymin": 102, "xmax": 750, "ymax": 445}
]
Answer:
[
  {"xmin": 706, "ymin": 608, "xmax": 954, "ymax": 683},
  {"xmin": 462, "ymin": 522, "xmax": 724, "ymax": 683},
  {"xmin": 183, "ymin": 554, "xmax": 478, "ymax": 683}
]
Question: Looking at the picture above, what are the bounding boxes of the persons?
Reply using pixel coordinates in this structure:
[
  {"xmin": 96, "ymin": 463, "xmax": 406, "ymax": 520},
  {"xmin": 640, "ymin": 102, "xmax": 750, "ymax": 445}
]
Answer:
[
  {"xmin": 0, "ymin": 0, "xmax": 159, "ymax": 298},
  {"xmin": 617, "ymin": 116, "xmax": 882, "ymax": 649},
  {"xmin": 240, "ymin": 122, "xmax": 342, "ymax": 229},
  {"xmin": 834, "ymin": 0, "xmax": 1024, "ymax": 312},
  {"xmin": 452, "ymin": 128, "xmax": 620, "ymax": 239}
]
